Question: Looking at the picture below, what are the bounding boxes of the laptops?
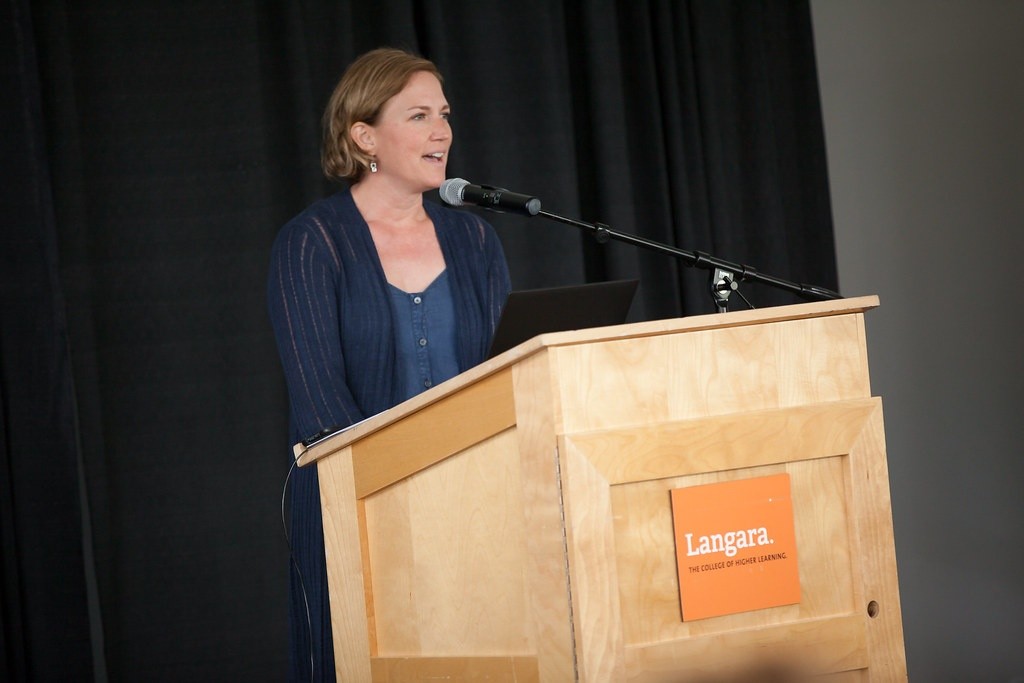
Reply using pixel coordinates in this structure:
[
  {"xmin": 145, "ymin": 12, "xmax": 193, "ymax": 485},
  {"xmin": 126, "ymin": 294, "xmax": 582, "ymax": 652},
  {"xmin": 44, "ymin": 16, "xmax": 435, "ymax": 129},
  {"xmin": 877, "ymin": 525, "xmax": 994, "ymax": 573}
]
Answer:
[{"xmin": 488, "ymin": 278, "xmax": 641, "ymax": 361}]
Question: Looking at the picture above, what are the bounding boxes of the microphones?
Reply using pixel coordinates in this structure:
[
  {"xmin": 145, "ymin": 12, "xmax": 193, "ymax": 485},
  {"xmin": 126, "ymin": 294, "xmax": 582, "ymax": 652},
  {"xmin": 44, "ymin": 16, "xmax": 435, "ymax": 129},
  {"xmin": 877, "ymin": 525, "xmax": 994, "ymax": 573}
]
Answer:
[{"xmin": 438, "ymin": 177, "xmax": 541, "ymax": 217}]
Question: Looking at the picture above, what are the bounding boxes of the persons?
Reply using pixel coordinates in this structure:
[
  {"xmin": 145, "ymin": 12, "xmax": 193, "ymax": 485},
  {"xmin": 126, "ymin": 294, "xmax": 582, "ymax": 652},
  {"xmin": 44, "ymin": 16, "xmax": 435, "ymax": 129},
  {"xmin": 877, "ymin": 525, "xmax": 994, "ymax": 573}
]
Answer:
[{"xmin": 267, "ymin": 48, "xmax": 511, "ymax": 682}]
